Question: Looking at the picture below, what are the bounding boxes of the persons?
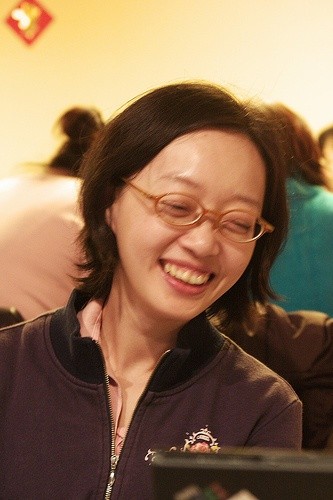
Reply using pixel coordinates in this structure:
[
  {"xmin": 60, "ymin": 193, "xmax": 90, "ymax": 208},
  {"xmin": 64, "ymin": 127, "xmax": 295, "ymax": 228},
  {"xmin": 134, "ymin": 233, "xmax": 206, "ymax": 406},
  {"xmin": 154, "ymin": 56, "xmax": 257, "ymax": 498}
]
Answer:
[
  {"xmin": 0, "ymin": 98, "xmax": 333, "ymax": 324},
  {"xmin": 0, "ymin": 81, "xmax": 302, "ymax": 500}
]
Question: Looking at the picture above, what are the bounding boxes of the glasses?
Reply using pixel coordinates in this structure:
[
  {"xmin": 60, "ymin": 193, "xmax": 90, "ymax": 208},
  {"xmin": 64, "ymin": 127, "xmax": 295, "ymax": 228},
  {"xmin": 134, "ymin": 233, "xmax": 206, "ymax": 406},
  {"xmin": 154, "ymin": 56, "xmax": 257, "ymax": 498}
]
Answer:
[{"xmin": 119, "ymin": 174, "xmax": 274, "ymax": 244}]
